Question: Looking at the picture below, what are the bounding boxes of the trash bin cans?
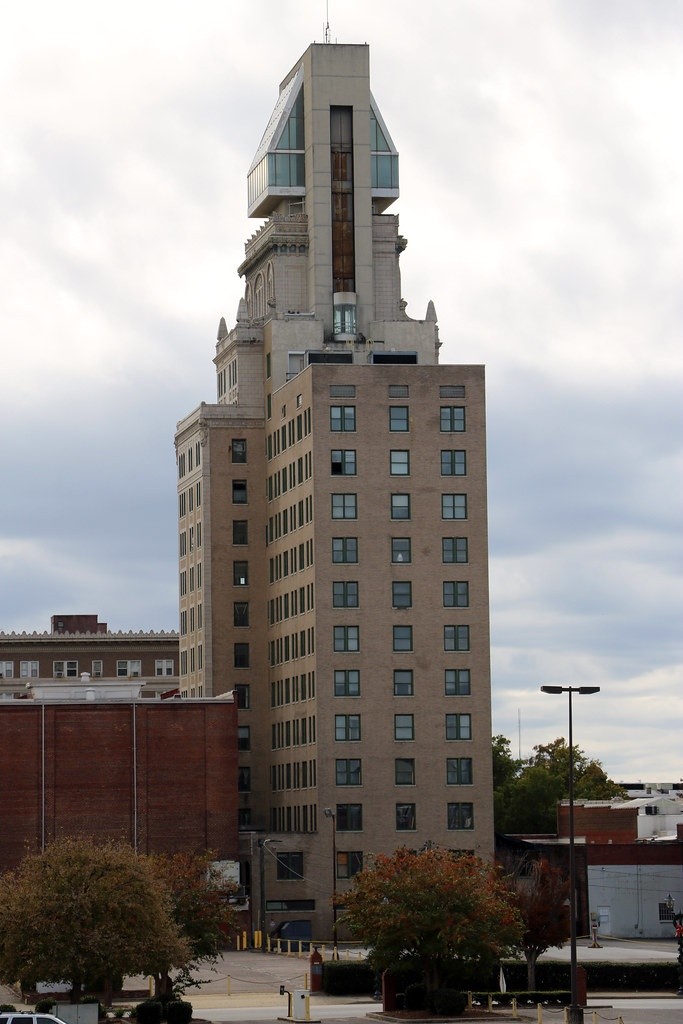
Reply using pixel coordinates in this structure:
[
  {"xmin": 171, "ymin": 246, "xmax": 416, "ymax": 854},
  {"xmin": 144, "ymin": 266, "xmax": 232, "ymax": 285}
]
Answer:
[
  {"xmin": 292, "ymin": 991, "xmax": 310, "ymax": 1020},
  {"xmin": 269, "ymin": 919, "xmax": 312, "ymax": 953}
]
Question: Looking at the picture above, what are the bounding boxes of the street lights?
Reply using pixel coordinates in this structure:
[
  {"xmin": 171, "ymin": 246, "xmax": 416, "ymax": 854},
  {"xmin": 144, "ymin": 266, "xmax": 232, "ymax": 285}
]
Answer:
[
  {"xmin": 539, "ymin": 685, "xmax": 601, "ymax": 1023},
  {"xmin": 324, "ymin": 807, "xmax": 340, "ymax": 962}
]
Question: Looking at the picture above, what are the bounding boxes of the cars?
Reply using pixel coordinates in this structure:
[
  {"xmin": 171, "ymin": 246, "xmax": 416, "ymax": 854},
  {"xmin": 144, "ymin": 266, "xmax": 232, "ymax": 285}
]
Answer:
[{"xmin": 0, "ymin": 1008, "xmax": 69, "ymax": 1024}]
